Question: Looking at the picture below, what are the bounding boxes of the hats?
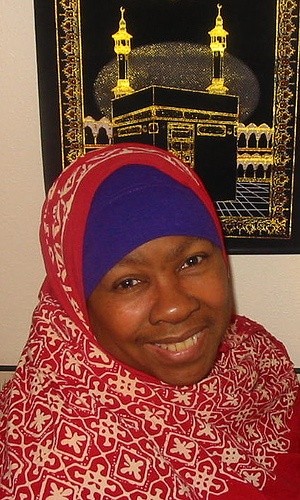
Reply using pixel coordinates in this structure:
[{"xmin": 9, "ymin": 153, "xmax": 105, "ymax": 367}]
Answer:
[{"xmin": 84, "ymin": 164, "xmax": 222, "ymax": 308}]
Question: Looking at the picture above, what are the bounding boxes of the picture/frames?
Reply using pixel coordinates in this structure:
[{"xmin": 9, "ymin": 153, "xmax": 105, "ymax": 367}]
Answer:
[{"xmin": 30, "ymin": 0, "xmax": 300, "ymax": 260}]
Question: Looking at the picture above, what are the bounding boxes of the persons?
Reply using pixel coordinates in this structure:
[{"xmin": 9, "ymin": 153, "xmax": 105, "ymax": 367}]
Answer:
[{"xmin": 0, "ymin": 144, "xmax": 300, "ymax": 500}]
